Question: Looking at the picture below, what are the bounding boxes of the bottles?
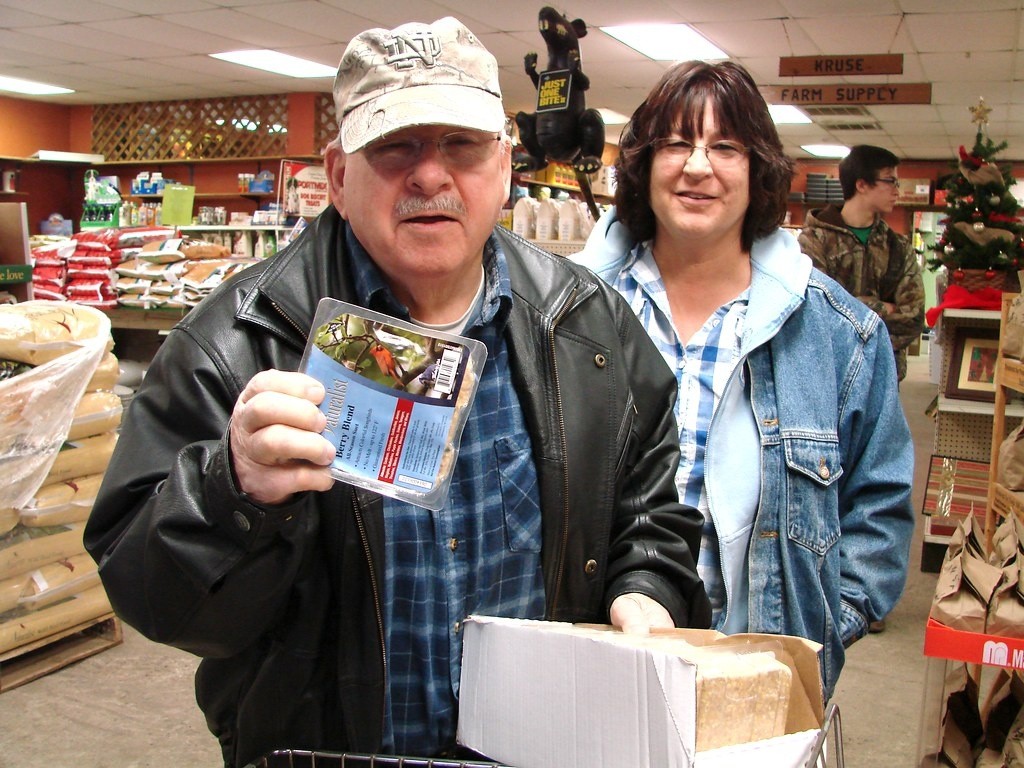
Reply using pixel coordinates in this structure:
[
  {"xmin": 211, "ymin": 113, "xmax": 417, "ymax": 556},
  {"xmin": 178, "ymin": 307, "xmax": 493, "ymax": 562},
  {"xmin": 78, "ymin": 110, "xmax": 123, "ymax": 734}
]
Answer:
[
  {"xmin": 118, "ymin": 201, "xmax": 161, "ymax": 227},
  {"xmin": 84, "ymin": 206, "xmax": 113, "ymax": 221},
  {"xmin": 198, "ymin": 206, "xmax": 227, "ymax": 225},
  {"xmin": 511, "ymin": 186, "xmax": 619, "ymax": 240}
]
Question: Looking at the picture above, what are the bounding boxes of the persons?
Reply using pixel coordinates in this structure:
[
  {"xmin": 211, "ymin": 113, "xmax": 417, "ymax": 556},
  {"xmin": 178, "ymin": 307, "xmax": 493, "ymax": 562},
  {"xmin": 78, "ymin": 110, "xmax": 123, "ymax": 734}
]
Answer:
[
  {"xmin": 563, "ymin": 59, "xmax": 913, "ymax": 703},
  {"xmin": 795, "ymin": 145, "xmax": 926, "ymax": 396},
  {"xmin": 84, "ymin": 14, "xmax": 714, "ymax": 768}
]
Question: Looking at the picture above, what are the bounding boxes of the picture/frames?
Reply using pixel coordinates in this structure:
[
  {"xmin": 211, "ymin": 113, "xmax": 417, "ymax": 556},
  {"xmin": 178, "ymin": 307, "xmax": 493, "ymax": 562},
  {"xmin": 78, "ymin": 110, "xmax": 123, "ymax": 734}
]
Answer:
[{"xmin": 945, "ymin": 326, "xmax": 1013, "ymax": 405}]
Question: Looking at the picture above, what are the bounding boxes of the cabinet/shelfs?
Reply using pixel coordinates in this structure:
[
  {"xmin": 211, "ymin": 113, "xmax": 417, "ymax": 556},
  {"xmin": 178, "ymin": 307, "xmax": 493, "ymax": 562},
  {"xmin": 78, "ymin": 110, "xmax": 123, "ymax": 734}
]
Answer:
[{"xmin": 919, "ymin": 309, "xmax": 1024, "ymax": 574}]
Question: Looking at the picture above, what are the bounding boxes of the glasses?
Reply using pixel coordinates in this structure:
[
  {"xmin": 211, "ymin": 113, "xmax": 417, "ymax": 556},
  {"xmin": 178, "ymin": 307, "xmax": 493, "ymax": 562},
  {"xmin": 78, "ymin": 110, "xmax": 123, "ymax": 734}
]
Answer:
[
  {"xmin": 870, "ymin": 178, "xmax": 900, "ymax": 188},
  {"xmin": 644, "ymin": 137, "xmax": 752, "ymax": 164},
  {"xmin": 360, "ymin": 129, "xmax": 502, "ymax": 170}
]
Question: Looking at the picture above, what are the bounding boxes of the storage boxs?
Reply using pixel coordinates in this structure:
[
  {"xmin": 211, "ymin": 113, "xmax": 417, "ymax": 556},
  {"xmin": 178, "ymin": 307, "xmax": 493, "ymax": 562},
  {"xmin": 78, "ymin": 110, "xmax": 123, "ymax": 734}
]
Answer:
[
  {"xmin": 456, "ymin": 613, "xmax": 826, "ymax": 768},
  {"xmin": 923, "ymin": 607, "xmax": 1024, "ymax": 670},
  {"xmin": 40, "ymin": 171, "xmax": 295, "ymax": 235}
]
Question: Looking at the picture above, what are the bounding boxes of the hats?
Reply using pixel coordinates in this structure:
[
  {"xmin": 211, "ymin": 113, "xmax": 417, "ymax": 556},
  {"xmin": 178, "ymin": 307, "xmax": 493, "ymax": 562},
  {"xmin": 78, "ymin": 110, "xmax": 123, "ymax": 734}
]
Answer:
[{"xmin": 332, "ymin": 16, "xmax": 506, "ymax": 154}]
